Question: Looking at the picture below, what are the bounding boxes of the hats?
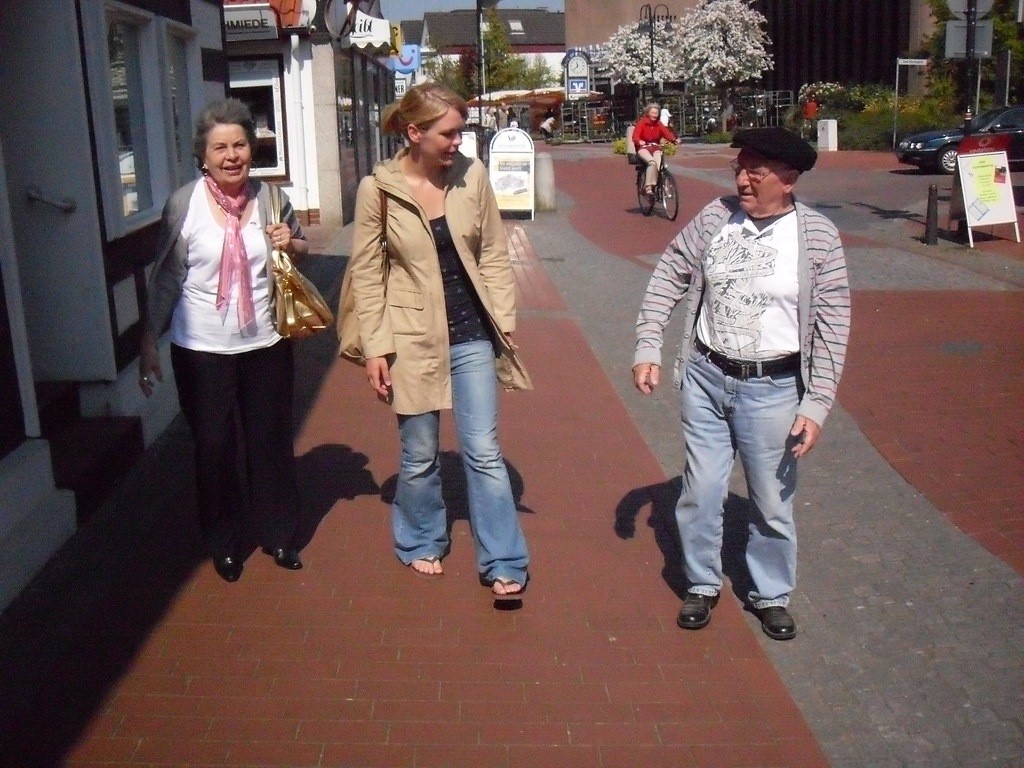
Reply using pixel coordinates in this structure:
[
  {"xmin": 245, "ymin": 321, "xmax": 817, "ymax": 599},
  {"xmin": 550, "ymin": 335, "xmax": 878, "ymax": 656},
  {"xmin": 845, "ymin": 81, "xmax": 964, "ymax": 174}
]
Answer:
[{"xmin": 729, "ymin": 125, "xmax": 817, "ymax": 175}]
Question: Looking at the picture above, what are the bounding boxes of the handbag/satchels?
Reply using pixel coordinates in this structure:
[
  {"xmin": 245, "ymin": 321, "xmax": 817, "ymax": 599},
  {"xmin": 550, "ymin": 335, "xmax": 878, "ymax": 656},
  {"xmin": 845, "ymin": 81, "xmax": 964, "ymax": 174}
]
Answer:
[
  {"xmin": 266, "ymin": 180, "xmax": 333, "ymax": 341},
  {"xmin": 337, "ymin": 172, "xmax": 387, "ymax": 367}
]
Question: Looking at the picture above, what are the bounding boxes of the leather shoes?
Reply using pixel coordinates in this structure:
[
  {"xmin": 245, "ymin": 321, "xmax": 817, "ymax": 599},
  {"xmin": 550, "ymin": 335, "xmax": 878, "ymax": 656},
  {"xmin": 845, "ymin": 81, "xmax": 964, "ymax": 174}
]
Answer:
[
  {"xmin": 677, "ymin": 591, "xmax": 720, "ymax": 629},
  {"xmin": 212, "ymin": 545, "xmax": 242, "ymax": 583},
  {"xmin": 261, "ymin": 543, "xmax": 303, "ymax": 569},
  {"xmin": 758, "ymin": 605, "xmax": 795, "ymax": 639}
]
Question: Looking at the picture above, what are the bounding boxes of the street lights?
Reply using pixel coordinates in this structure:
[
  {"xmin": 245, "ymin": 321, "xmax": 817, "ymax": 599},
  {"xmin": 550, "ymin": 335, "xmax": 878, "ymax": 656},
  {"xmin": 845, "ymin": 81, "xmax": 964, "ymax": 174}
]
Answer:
[{"xmin": 638, "ymin": 2, "xmax": 674, "ymax": 101}]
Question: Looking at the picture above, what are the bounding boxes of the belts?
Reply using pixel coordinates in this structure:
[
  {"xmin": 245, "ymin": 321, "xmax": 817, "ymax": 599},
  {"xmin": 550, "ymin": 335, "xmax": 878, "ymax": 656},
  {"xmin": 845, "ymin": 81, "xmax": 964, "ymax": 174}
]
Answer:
[{"xmin": 695, "ymin": 336, "xmax": 799, "ymax": 378}]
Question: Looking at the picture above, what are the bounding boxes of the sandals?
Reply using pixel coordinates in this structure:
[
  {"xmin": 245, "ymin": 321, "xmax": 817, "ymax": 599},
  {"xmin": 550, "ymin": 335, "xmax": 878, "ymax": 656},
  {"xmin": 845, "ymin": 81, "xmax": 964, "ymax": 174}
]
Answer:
[
  {"xmin": 493, "ymin": 577, "xmax": 523, "ymax": 602},
  {"xmin": 410, "ymin": 557, "xmax": 445, "ymax": 578}
]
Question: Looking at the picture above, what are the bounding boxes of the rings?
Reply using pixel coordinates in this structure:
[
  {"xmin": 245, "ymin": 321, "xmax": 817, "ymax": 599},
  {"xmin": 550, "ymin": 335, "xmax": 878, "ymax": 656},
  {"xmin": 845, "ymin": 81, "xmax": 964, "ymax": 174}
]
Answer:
[
  {"xmin": 144, "ymin": 377, "xmax": 149, "ymax": 380},
  {"xmin": 278, "ymin": 236, "xmax": 281, "ymax": 246}
]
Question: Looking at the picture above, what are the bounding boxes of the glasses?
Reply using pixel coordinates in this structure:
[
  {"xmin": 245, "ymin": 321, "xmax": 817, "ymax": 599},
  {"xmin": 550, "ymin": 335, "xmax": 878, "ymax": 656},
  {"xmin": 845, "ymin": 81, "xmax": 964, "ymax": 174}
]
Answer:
[{"xmin": 729, "ymin": 157, "xmax": 780, "ymax": 181}]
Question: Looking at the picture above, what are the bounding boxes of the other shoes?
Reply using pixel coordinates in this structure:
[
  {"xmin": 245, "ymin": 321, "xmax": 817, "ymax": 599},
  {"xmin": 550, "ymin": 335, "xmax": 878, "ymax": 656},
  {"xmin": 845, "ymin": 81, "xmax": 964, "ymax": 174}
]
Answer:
[{"xmin": 640, "ymin": 185, "xmax": 655, "ymax": 196}]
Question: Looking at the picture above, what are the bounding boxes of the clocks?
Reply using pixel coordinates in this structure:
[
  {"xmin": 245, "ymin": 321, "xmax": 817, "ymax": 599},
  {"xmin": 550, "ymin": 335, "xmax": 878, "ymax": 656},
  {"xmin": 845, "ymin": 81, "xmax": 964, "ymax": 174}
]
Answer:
[{"xmin": 570, "ymin": 57, "xmax": 586, "ymax": 76}]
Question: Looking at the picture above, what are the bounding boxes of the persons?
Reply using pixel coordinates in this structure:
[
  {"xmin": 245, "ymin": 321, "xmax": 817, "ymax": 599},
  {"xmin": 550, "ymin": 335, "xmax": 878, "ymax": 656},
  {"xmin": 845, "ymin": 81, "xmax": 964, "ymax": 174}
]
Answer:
[
  {"xmin": 632, "ymin": 126, "xmax": 851, "ymax": 639},
  {"xmin": 482, "ymin": 101, "xmax": 509, "ymax": 148},
  {"xmin": 351, "ymin": 82, "xmax": 534, "ymax": 600},
  {"xmin": 632, "ymin": 103, "xmax": 681, "ymax": 194},
  {"xmin": 660, "ymin": 103, "xmax": 673, "ymax": 127},
  {"xmin": 541, "ymin": 116, "xmax": 556, "ymax": 138},
  {"xmin": 139, "ymin": 98, "xmax": 310, "ymax": 582}
]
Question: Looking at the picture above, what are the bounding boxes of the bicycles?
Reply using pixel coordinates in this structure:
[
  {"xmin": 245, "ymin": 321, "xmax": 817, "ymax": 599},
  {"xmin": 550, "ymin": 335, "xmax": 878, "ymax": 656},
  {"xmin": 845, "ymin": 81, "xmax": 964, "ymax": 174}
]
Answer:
[{"xmin": 635, "ymin": 140, "xmax": 680, "ymax": 221}]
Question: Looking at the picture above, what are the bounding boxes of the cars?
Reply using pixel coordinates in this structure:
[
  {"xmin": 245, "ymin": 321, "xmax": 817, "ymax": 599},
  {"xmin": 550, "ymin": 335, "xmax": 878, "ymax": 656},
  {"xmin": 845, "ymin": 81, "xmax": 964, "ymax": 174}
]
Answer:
[
  {"xmin": 685, "ymin": 92, "xmax": 790, "ymax": 134},
  {"xmin": 896, "ymin": 105, "xmax": 1024, "ymax": 175},
  {"xmin": 563, "ymin": 98, "xmax": 636, "ymax": 135}
]
